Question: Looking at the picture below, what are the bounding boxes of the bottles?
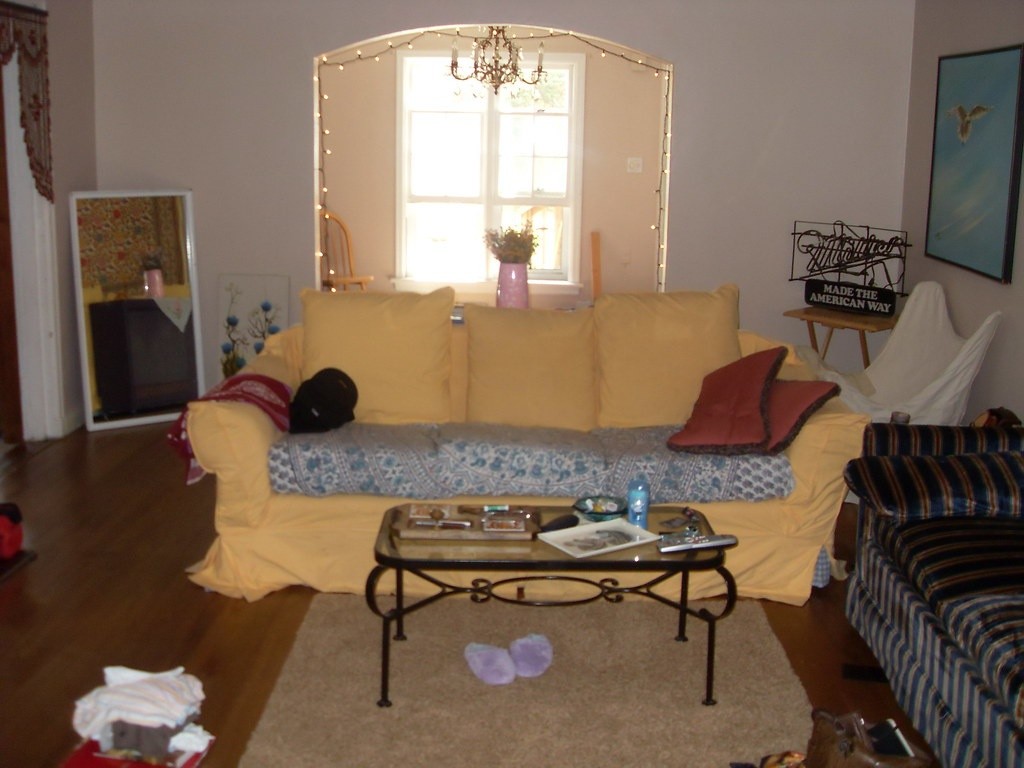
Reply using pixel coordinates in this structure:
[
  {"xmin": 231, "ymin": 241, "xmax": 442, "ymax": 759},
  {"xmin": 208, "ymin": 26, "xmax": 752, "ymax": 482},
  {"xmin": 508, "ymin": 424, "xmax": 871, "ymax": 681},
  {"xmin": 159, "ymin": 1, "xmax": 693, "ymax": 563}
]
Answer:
[
  {"xmin": 629, "ymin": 482, "xmax": 649, "ymax": 530},
  {"xmin": 144, "ymin": 261, "xmax": 163, "ymax": 297}
]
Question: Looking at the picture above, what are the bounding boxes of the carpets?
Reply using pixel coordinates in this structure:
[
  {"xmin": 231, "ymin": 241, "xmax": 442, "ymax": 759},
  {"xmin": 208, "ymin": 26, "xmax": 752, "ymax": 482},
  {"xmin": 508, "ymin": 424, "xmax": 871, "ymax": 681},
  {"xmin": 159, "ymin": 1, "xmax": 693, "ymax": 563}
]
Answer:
[{"xmin": 234, "ymin": 596, "xmax": 814, "ymax": 768}]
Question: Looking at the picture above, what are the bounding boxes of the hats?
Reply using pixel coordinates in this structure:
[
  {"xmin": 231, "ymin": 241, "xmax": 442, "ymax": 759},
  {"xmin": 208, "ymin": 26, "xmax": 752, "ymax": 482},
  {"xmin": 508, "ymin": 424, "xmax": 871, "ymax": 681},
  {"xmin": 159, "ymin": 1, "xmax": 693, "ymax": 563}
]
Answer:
[{"xmin": 284, "ymin": 367, "xmax": 360, "ymax": 434}]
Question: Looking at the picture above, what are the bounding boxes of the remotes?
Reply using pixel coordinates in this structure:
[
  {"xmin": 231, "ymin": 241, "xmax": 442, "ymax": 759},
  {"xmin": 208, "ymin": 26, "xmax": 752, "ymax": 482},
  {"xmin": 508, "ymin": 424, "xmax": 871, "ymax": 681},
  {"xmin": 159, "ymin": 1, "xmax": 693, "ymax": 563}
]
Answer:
[{"xmin": 656, "ymin": 534, "xmax": 738, "ymax": 553}]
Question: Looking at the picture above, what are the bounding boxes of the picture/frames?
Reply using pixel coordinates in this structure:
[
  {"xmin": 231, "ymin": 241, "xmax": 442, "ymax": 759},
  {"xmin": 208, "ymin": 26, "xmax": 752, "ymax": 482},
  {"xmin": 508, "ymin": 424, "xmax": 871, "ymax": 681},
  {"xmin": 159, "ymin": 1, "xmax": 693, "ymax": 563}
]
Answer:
[{"xmin": 923, "ymin": 43, "xmax": 1024, "ymax": 284}]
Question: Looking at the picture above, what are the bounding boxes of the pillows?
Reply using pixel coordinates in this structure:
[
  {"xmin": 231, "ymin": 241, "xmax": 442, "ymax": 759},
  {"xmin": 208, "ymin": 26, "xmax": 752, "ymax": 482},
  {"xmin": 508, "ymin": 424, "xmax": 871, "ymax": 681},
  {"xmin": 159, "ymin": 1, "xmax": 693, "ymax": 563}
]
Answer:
[
  {"xmin": 844, "ymin": 452, "xmax": 1023, "ymax": 523},
  {"xmin": 298, "ymin": 285, "xmax": 457, "ymax": 425},
  {"xmin": 747, "ymin": 379, "xmax": 842, "ymax": 451},
  {"xmin": 591, "ymin": 286, "xmax": 746, "ymax": 434},
  {"xmin": 667, "ymin": 345, "xmax": 789, "ymax": 453}
]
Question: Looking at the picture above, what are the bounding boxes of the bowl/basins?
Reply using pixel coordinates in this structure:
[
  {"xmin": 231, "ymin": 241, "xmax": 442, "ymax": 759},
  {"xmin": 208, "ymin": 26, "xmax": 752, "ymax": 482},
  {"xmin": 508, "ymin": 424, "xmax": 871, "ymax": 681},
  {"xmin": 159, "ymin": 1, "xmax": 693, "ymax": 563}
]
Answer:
[{"xmin": 572, "ymin": 495, "xmax": 627, "ymax": 521}]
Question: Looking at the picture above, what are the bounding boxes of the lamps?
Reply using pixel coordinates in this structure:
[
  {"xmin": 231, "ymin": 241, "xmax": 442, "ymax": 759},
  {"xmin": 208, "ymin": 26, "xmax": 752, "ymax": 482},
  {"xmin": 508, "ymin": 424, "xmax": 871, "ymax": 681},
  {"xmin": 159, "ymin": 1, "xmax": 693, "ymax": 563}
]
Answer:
[{"xmin": 450, "ymin": 24, "xmax": 559, "ymax": 105}]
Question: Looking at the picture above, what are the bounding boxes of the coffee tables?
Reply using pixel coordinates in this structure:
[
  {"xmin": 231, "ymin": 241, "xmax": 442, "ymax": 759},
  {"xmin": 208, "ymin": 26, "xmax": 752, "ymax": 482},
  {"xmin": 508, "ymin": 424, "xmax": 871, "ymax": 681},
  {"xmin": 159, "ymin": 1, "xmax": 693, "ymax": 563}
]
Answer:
[{"xmin": 365, "ymin": 504, "xmax": 737, "ymax": 706}]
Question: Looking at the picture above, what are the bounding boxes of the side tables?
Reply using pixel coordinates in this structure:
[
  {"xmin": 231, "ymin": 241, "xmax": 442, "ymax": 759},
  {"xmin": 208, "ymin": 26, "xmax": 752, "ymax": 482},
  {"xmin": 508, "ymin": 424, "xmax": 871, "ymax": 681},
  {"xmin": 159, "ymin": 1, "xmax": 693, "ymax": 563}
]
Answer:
[{"xmin": 785, "ymin": 305, "xmax": 901, "ymax": 371}]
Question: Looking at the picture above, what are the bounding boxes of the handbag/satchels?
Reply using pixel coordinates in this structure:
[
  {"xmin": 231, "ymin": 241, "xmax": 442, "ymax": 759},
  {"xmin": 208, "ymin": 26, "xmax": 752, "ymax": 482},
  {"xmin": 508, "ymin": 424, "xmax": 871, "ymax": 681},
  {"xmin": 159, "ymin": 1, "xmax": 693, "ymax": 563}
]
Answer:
[{"xmin": 805, "ymin": 709, "xmax": 943, "ymax": 768}]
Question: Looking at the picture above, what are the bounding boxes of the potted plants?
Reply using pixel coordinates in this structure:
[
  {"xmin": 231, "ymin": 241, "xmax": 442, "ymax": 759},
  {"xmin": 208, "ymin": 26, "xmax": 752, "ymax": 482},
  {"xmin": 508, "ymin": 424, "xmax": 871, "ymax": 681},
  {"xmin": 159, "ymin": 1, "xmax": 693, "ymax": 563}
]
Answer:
[
  {"xmin": 142, "ymin": 253, "xmax": 166, "ymax": 294},
  {"xmin": 484, "ymin": 220, "xmax": 545, "ymax": 311}
]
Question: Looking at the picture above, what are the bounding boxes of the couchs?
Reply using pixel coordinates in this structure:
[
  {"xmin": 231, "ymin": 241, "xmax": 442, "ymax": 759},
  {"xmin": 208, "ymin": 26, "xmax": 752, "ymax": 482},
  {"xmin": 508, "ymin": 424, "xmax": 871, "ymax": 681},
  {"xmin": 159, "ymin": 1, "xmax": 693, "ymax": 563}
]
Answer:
[
  {"xmin": 841, "ymin": 420, "xmax": 1024, "ymax": 768},
  {"xmin": 186, "ymin": 306, "xmax": 865, "ymax": 603}
]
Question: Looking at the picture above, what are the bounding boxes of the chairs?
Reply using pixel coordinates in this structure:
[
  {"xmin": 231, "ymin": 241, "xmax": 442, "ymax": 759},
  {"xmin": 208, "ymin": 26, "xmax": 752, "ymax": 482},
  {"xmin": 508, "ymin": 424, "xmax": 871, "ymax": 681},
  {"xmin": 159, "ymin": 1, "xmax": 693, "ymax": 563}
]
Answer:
[{"xmin": 320, "ymin": 208, "xmax": 375, "ymax": 292}]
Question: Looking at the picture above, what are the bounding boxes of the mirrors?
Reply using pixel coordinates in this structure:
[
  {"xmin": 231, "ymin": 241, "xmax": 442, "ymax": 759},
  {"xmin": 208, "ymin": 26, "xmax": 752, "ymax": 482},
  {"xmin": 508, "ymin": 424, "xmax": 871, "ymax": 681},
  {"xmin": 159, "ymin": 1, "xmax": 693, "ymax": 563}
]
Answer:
[{"xmin": 67, "ymin": 190, "xmax": 208, "ymax": 430}]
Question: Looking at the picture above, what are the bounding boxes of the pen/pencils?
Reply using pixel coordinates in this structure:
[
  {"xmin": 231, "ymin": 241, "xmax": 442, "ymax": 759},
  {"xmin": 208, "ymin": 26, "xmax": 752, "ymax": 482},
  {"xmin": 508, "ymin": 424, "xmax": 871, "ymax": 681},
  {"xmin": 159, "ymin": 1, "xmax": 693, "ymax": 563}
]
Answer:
[{"xmin": 417, "ymin": 520, "xmax": 472, "ymax": 529}]
